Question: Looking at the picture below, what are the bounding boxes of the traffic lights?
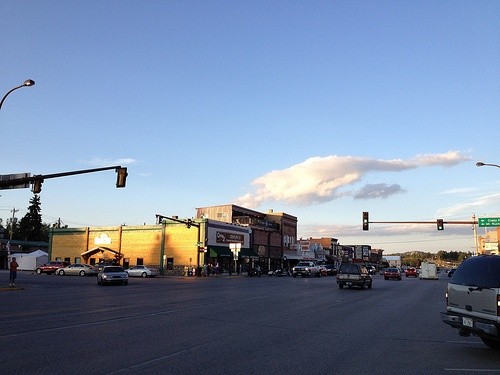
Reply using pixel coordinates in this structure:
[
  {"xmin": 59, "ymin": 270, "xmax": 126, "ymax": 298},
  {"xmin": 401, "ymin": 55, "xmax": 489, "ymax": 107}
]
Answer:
[
  {"xmin": 187, "ymin": 221, "xmax": 191, "ymax": 228},
  {"xmin": 437, "ymin": 219, "xmax": 444, "ymax": 230},
  {"xmin": 115, "ymin": 167, "xmax": 128, "ymax": 188},
  {"xmin": 363, "ymin": 212, "xmax": 368, "ymax": 230},
  {"xmin": 32, "ymin": 175, "xmax": 44, "ymax": 193},
  {"xmin": 157, "ymin": 216, "xmax": 162, "ymax": 224}
]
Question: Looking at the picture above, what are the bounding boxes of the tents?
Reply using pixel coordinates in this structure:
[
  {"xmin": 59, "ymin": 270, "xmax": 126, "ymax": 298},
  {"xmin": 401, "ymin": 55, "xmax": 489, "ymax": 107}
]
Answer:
[{"xmin": 8, "ymin": 249, "xmax": 48, "ymax": 271}]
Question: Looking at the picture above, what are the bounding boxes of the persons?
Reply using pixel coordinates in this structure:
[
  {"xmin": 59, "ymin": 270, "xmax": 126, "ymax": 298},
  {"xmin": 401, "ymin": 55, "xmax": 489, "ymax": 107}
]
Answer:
[
  {"xmin": 214, "ymin": 263, "xmax": 221, "ymax": 279},
  {"xmin": 202, "ymin": 263, "xmax": 212, "ymax": 278},
  {"xmin": 9, "ymin": 257, "xmax": 19, "ymax": 287}
]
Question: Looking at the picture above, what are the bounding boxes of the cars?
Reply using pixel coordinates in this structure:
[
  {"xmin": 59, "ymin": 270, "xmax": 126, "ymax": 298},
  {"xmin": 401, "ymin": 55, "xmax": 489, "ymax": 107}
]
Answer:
[
  {"xmin": 97, "ymin": 265, "xmax": 128, "ymax": 285},
  {"xmin": 124, "ymin": 265, "xmax": 159, "ymax": 277},
  {"xmin": 56, "ymin": 264, "xmax": 100, "ymax": 276},
  {"xmin": 366, "ymin": 266, "xmax": 376, "ymax": 275},
  {"xmin": 380, "ymin": 268, "xmax": 387, "ymax": 275},
  {"xmin": 318, "ymin": 269, "xmax": 327, "ymax": 276},
  {"xmin": 320, "ymin": 265, "xmax": 339, "ymax": 275}
]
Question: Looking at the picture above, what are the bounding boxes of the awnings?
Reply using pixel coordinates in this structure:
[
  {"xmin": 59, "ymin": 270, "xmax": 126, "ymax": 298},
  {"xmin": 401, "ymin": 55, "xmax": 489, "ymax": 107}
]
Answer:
[
  {"xmin": 239, "ymin": 248, "xmax": 260, "ymax": 258},
  {"xmin": 206, "ymin": 245, "xmax": 232, "ymax": 257}
]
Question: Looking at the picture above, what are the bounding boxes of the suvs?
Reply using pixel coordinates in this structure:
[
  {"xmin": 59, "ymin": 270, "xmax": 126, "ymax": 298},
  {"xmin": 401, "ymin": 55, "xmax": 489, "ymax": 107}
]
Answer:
[
  {"xmin": 35, "ymin": 261, "xmax": 71, "ymax": 275},
  {"xmin": 405, "ymin": 268, "xmax": 417, "ymax": 277},
  {"xmin": 439, "ymin": 253, "xmax": 500, "ymax": 347},
  {"xmin": 384, "ymin": 267, "xmax": 401, "ymax": 280},
  {"xmin": 293, "ymin": 261, "xmax": 319, "ymax": 278},
  {"xmin": 336, "ymin": 263, "xmax": 373, "ymax": 289},
  {"xmin": 419, "ymin": 261, "xmax": 439, "ymax": 279}
]
